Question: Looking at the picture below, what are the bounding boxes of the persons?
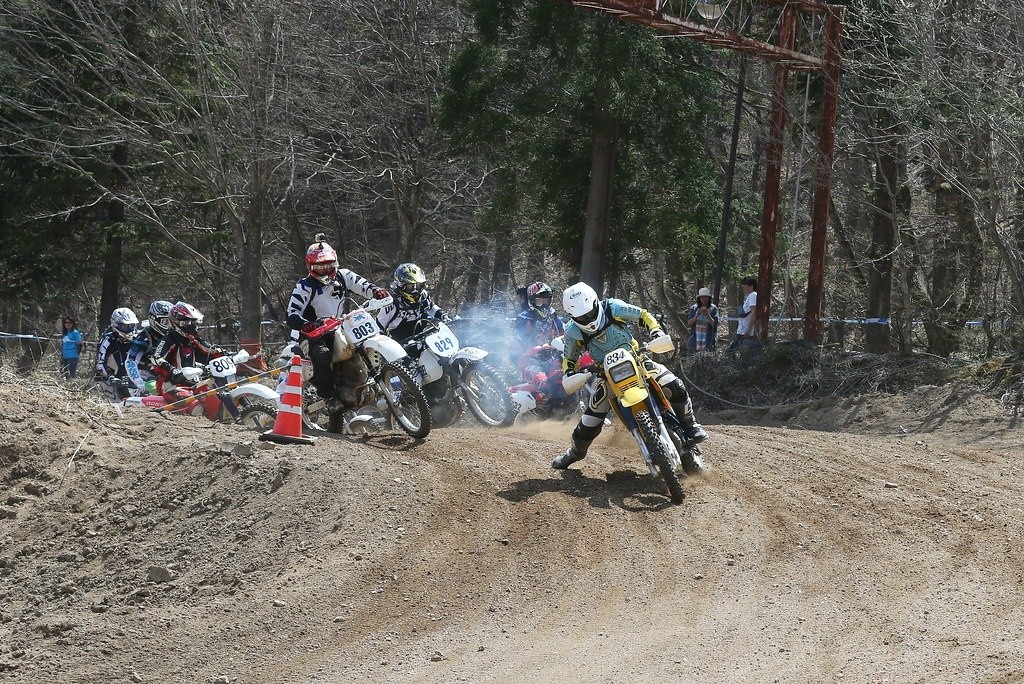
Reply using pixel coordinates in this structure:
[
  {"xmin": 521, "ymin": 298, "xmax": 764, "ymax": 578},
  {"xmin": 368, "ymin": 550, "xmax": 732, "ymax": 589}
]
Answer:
[
  {"xmin": 551, "ymin": 281, "xmax": 709, "ymax": 470},
  {"xmin": 726, "ymin": 276, "xmax": 757, "ymax": 351},
  {"xmin": 374, "ymin": 263, "xmax": 452, "ymax": 411},
  {"xmin": 688, "ymin": 287, "xmax": 719, "ymax": 351},
  {"xmin": 511, "ymin": 281, "xmax": 568, "ymax": 421},
  {"xmin": 286, "ymin": 242, "xmax": 388, "ymax": 413},
  {"xmin": 60, "ymin": 316, "xmax": 82, "ymax": 381},
  {"xmin": 94, "ymin": 300, "xmax": 229, "ymax": 416}
]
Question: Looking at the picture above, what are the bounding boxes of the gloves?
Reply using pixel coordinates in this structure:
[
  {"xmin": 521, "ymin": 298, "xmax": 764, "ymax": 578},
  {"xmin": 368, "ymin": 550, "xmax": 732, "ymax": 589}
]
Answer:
[
  {"xmin": 110, "ymin": 377, "xmax": 122, "ymax": 387},
  {"xmin": 443, "ymin": 313, "xmax": 460, "ymax": 321},
  {"xmin": 373, "ymin": 288, "xmax": 389, "ymax": 299},
  {"xmin": 171, "ymin": 367, "xmax": 184, "ymax": 382},
  {"xmin": 302, "ymin": 321, "xmax": 315, "ymax": 333}
]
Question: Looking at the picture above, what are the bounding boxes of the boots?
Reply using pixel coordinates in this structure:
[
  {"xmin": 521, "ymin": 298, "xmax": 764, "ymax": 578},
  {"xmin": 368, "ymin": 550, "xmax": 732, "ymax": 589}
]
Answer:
[
  {"xmin": 670, "ymin": 396, "xmax": 709, "ymax": 443},
  {"xmin": 552, "ymin": 433, "xmax": 593, "ymax": 469}
]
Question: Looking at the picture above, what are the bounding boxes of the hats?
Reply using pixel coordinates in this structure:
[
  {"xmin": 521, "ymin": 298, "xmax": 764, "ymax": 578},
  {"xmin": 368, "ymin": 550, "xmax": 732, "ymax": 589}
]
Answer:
[{"xmin": 696, "ymin": 288, "xmax": 712, "ymax": 298}]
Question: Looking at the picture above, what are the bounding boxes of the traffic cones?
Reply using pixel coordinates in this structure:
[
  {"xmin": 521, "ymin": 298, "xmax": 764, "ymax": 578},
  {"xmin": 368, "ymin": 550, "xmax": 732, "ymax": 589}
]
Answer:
[{"xmin": 259, "ymin": 346, "xmax": 315, "ymax": 445}]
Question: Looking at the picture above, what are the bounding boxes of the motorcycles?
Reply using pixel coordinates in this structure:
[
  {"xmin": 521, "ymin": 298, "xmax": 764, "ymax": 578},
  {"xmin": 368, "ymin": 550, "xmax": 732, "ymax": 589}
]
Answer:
[
  {"xmin": 561, "ymin": 333, "xmax": 702, "ymax": 506},
  {"xmin": 341, "ymin": 314, "xmax": 515, "ymax": 434},
  {"xmin": 96, "ymin": 343, "xmax": 279, "ymax": 431},
  {"xmin": 301, "ymin": 292, "xmax": 431, "ymax": 440},
  {"xmin": 508, "ymin": 332, "xmax": 615, "ymax": 422}
]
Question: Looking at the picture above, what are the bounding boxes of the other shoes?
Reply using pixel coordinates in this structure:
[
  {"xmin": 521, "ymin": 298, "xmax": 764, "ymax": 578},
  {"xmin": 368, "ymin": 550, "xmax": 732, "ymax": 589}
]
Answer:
[{"xmin": 323, "ymin": 395, "xmax": 344, "ymax": 413}]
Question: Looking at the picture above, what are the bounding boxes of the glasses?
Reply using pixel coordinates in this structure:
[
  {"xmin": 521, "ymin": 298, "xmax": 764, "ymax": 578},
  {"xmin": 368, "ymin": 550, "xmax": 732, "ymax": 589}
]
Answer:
[
  {"xmin": 118, "ymin": 323, "xmax": 134, "ymax": 332},
  {"xmin": 533, "ymin": 297, "xmax": 551, "ymax": 308},
  {"xmin": 402, "ymin": 283, "xmax": 424, "ymax": 294},
  {"xmin": 159, "ymin": 317, "xmax": 168, "ymax": 327},
  {"xmin": 66, "ymin": 322, "xmax": 72, "ymax": 324},
  {"xmin": 179, "ymin": 319, "xmax": 200, "ymax": 329}
]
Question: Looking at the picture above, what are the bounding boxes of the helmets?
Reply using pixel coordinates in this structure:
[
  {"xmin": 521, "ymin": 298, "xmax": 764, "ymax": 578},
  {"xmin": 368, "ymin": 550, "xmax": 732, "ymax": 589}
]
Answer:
[
  {"xmin": 168, "ymin": 301, "xmax": 203, "ymax": 340},
  {"xmin": 393, "ymin": 263, "xmax": 427, "ymax": 303},
  {"xmin": 527, "ymin": 281, "xmax": 553, "ymax": 318},
  {"xmin": 562, "ymin": 281, "xmax": 602, "ymax": 333},
  {"xmin": 111, "ymin": 307, "xmax": 139, "ymax": 342},
  {"xmin": 306, "ymin": 242, "xmax": 339, "ymax": 285},
  {"xmin": 148, "ymin": 300, "xmax": 174, "ymax": 336}
]
